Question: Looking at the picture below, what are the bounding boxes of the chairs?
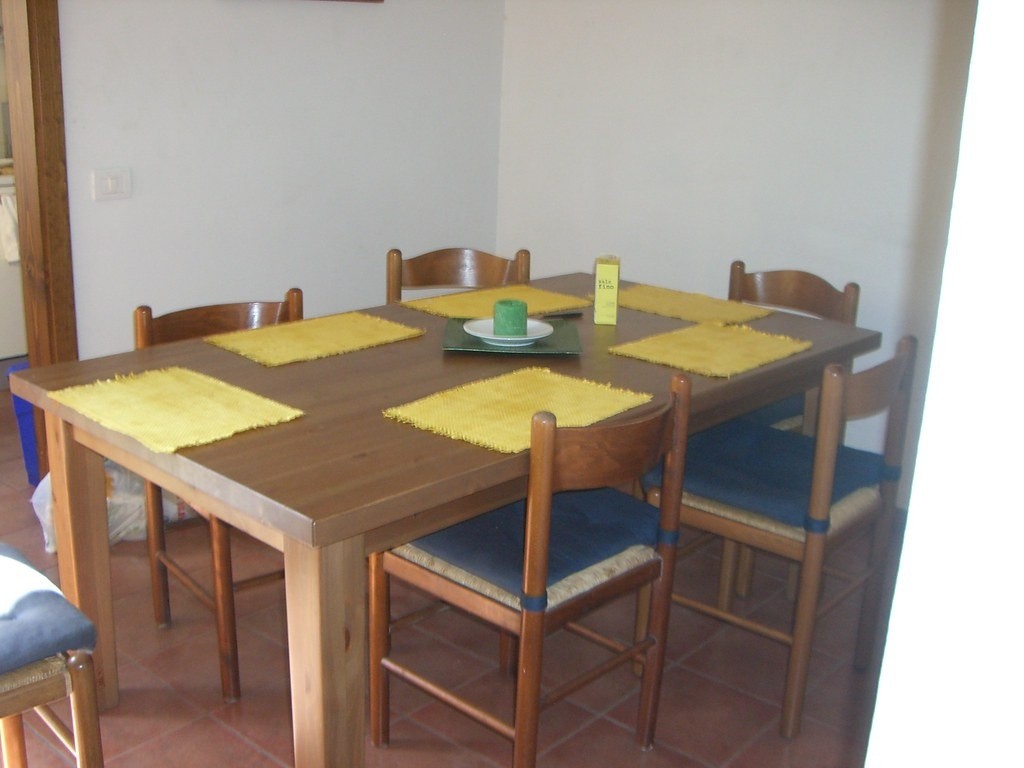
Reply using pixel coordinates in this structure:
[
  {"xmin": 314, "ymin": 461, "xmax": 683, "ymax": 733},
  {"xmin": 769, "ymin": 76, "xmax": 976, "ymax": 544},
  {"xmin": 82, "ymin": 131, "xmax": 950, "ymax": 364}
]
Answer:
[
  {"xmin": 386, "ymin": 248, "xmax": 530, "ymax": 304},
  {"xmin": 0, "ymin": 543, "xmax": 105, "ymax": 768},
  {"xmin": 633, "ymin": 336, "xmax": 920, "ymax": 742},
  {"xmin": 366, "ymin": 374, "xmax": 691, "ymax": 768},
  {"xmin": 135, "ymin": 288, "xmax": 304, "ymax": 703},
  {"xmin": 728, "ymin": 260, "xmax": 860, "ymax": 601}
]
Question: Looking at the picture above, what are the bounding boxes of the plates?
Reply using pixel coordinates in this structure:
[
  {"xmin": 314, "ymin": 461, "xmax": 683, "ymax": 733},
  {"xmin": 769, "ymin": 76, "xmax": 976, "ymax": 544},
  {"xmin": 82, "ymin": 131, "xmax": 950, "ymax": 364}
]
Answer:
[
  {"xmin": 463, "ymin": 318, "xmax": 554, "ymax": 346},
  {"xmin": 442, "ymin": 318, "xmax": 583, "ymax": 354}
]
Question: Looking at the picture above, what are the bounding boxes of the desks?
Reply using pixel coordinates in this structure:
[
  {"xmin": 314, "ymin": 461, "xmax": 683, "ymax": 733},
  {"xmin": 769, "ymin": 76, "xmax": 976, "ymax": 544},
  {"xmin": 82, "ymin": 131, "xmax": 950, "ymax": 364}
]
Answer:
[{"xmin": 8, "ymin": 273, "xmax": 882, "ymax": 768}]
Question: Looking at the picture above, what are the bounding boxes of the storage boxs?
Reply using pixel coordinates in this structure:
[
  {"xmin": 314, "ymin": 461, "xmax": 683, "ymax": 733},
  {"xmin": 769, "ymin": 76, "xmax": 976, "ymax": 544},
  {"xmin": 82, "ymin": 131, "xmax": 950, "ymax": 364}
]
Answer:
[{"xmin": 592, "ymin": 258, "xmax": 620, "ymax": 325}]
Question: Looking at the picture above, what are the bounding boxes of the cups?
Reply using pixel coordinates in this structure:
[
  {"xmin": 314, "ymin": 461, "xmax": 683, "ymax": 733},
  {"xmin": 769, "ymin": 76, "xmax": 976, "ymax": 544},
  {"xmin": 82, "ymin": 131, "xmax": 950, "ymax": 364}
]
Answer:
[{"xmin": 493, "ymin": 299, "xmax": 528, "ymax": 337}]
